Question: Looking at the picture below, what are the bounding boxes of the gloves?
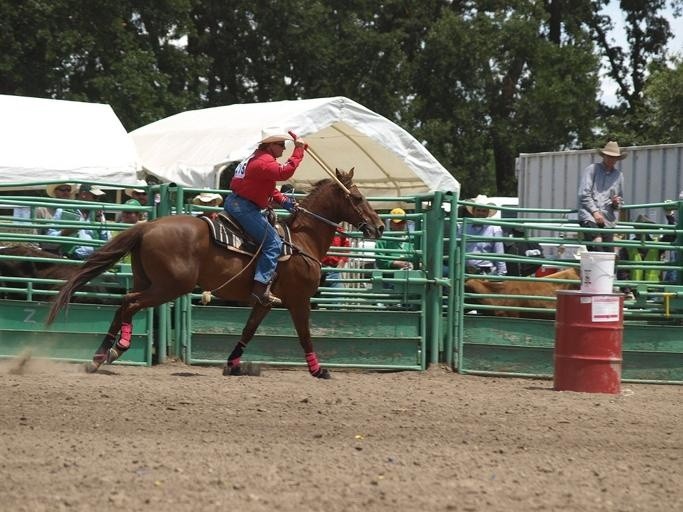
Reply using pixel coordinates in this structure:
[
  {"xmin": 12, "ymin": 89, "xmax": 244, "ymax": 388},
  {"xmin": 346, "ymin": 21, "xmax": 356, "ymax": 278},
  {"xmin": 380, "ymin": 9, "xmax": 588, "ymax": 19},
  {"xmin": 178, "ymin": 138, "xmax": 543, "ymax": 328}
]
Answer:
[{"xmin": 282, "ymin": 197, "xmax": 298, "ymax": 211}]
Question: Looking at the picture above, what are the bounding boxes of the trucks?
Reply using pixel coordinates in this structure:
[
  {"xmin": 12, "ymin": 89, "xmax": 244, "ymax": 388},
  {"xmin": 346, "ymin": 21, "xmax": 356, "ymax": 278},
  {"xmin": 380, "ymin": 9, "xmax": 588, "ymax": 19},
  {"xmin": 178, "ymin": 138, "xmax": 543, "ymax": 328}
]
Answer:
[{"xmin": 512, "ymin": 143, "xmax": 681, "ymax": 259}]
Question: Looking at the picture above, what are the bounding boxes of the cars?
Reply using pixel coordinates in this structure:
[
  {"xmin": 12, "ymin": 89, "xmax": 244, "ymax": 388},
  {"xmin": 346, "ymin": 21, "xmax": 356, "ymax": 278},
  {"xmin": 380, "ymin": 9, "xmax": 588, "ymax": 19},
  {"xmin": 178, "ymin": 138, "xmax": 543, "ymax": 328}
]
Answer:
[{"xmin": 343, "ymin": 195, "xmax": 519, "ymax": 283}]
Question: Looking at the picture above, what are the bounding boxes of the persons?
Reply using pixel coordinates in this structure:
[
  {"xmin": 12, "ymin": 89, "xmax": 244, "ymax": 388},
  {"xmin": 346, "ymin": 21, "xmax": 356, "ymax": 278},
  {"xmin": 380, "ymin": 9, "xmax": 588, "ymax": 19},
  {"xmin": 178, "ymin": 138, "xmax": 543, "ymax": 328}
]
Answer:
[
  {"xmin": 224, "ymin": 127, "xmax": 305, "ymax": 305},
  {"xmin": 577, "ymin": 141, "xmax": 627, "ymax": 251}
]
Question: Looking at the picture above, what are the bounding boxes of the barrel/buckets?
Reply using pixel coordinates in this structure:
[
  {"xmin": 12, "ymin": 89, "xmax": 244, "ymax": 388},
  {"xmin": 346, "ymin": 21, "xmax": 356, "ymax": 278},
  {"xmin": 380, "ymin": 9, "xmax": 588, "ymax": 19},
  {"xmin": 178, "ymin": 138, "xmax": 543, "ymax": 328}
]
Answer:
[
  {"xmin": 535, "ymin": 242, "xmax": 559, "ymax": 278},
  {"xmin": 552, "ymin": 286, "xmax": 627, "ymax": 396},
  {"xmin": 559, "ymin": 244, "xmax": 587, "ymax": 273},
  {"xmin": 578, "ymin": 250, "xmax": 617, "ymax": 295}
]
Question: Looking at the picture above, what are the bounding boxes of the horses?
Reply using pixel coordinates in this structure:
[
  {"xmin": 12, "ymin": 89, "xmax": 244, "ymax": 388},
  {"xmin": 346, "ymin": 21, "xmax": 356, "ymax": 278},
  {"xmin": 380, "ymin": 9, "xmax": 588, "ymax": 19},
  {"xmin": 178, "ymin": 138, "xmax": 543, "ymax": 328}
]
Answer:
[
  {"xmin": 47, "ymin": 167, "xmax": 385, "ymax": 379},
  {"xmin": 0, "ymin": 245, "xmax": 127, "ymax": 305},
  {"xmin": 464, "ymin": 267, "xmax": 582, "ymax": 318}
]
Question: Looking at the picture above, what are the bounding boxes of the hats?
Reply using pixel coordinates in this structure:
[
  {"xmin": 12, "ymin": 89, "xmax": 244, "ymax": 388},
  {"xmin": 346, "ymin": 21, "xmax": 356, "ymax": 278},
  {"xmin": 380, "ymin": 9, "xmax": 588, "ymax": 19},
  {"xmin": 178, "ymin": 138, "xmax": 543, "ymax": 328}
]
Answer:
[
  {"xmin": 193, "ymin": 187, "xmax": 223, "ymax": 205},
  {"xmin": 464, "ymin": 194, "xmax": 496, "ymax": 216},
  {"xmin": 79, "ymin": 183, "xmax": 106, "ymax": 194},
  {"xmin": 257, "ymin": 125, "xmax": 294, "ymax": 144},
  {"xmin": 598, "ymin": 141, "xmax": 628, "ymax": 160},
  {"xmin": 46, "ymin": 176, "xmax": 76, "ymax": 198},
  {"xmin": 126, "ymin": 179, "xmax": 148, "ymax": 196},
  {"xmin": 389, "ymin": 208, "xmax": 406, "ymax": 223}
]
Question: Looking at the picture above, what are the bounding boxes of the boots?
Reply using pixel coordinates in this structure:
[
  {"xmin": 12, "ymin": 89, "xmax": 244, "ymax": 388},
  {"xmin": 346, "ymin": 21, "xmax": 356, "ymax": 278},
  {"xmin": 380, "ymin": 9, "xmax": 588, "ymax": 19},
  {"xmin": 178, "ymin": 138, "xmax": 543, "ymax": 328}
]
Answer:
[{"xmin": 252, "ymin": 271, "xmax": 282, "ymax": 305}]
